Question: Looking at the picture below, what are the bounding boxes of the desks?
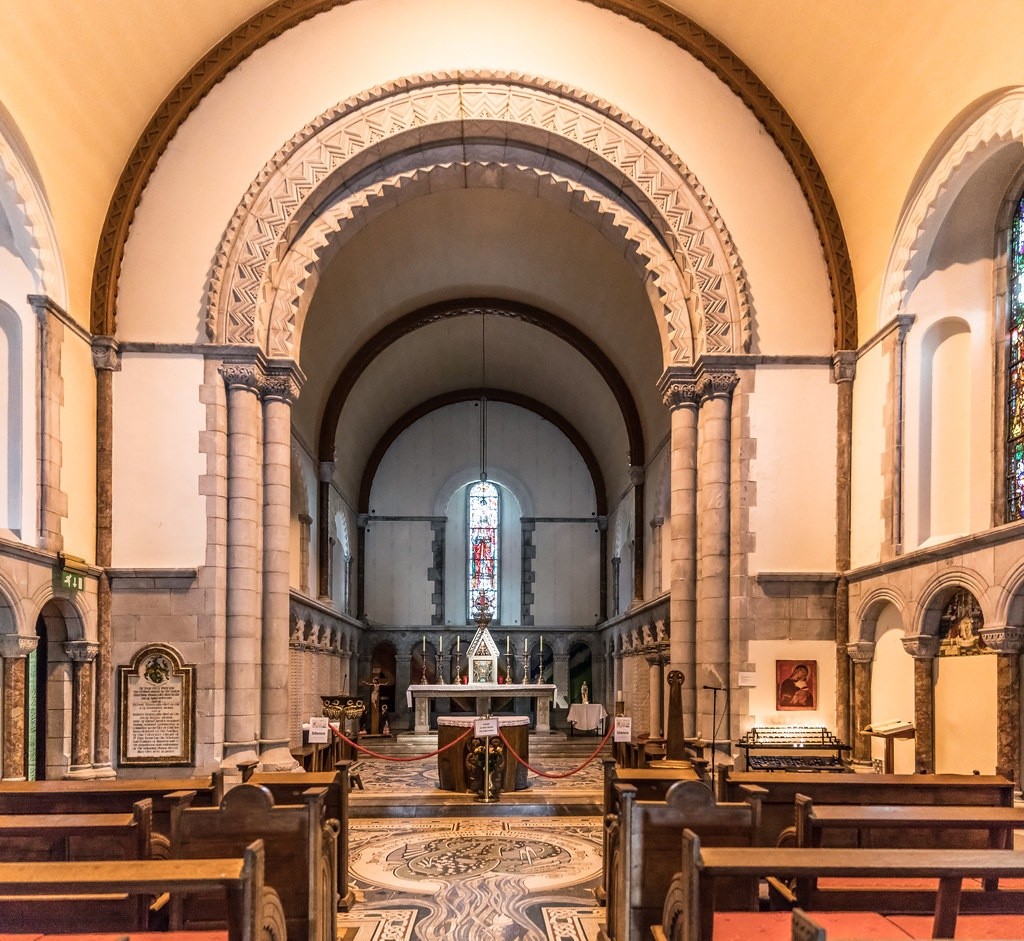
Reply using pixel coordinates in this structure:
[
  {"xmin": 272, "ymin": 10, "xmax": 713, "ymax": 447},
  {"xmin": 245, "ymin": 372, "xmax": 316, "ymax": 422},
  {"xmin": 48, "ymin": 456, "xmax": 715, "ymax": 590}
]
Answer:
[
  {"xmin": 438, "ymin": 716, "xmax": 530, "ymax": 793},
  {"xmin": 410, "ymin": 684, "xmax": 557, "ymax": 734},
  {"xmin": 570, "ymin": 704, "xmax": 603, "ymax": 739}
]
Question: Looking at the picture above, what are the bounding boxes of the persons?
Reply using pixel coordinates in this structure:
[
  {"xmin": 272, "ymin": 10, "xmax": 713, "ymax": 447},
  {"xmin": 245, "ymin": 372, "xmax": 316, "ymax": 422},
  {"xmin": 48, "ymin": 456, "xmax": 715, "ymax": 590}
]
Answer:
[
  {"xmin": 362, "ymin": 679, "xmax": 390, "ymax": 709},
  {"xmin": 581, "ymin": 681, "xmax": 589, "ymax": 704},
  {"xmin": 467, "ymin": 737, "xmax": 505, "ymax": 795},
  {"xmin": 380, "ymin": 704, "xmax": 391, "ymax": 734}
]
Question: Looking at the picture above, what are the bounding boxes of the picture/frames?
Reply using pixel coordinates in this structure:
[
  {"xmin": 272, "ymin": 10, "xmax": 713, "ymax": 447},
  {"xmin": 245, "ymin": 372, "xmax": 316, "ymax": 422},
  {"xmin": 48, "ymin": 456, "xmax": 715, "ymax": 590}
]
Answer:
[{"xmin": 776, "ymin": 660, "xmax": 817, "ymax": 711}]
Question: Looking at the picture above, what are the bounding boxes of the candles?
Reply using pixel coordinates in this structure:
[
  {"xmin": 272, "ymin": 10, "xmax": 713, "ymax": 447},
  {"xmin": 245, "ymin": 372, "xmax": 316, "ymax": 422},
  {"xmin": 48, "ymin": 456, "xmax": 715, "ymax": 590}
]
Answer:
[
  {"xmin": 506, "ymin": 636, "xmax": 509, "ymax": 652},
  {"xmin": 540, "ymin": 636, "xmax": 543, "ymax": 651},
  {"xmin": 525, "ymin": 638, "xmax": 528, "ymax": 651},
  {"xmin": 482, "ymin": 641, "xmax": 484, "ymax": 647},
  {"xmin": 440, "ymin": 636, "xmax": 442, "ymax": 652},
  {"xmin": 457, "ymin": 636, "xmax": 460, "ymax": 651},
  {"xmin": 422, "ymin": 636, "xmax": 425, "ymax": 652}
]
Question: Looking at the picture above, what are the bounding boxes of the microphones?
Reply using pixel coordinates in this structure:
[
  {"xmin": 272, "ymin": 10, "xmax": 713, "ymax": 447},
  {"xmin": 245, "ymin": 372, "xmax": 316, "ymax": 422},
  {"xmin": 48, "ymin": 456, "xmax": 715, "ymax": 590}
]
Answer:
[
  {"xmin": 703, "ymin": 686, "xmax": 726, "ymax": 690},
  {"xmin": 342, "ymin": 674, "xmax": 347, "ymax": 695}
]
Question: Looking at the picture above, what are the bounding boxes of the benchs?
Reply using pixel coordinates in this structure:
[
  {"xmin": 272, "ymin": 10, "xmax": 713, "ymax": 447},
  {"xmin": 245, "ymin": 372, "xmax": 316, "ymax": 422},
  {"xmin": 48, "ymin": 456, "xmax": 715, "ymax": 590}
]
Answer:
[
  {"xmin": 0, "ymin": 758, "xmax": 354, "ymax": 941},
  {"xmin": 599, "ymin": 757, "xmax": 1024, "ymax": 941}
]
